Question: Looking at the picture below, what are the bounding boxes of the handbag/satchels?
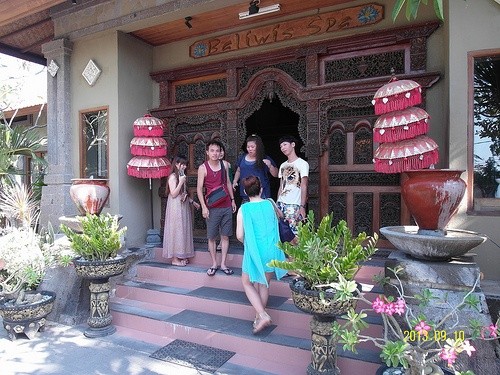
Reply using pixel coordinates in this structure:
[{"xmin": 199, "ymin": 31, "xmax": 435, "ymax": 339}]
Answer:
[
  {"xmin": 268, "ymin": 198, "xmax": 295, "ymax": 243},
  {"xmin": 205, "ymin": 185, "xmax": 229, "ymax": 208}
]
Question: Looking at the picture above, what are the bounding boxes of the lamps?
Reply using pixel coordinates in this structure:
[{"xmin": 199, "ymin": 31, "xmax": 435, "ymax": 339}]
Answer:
[
  {"xmin": 185, "ymin": 16, "xmax": 192, "ymax": 29},
  {"xmin": 239, "ymin": 0, "xmax": 280, "ymax": 19},
  {"xmin": 249, "ymin": 0, "xmax": 261, "ymax": 15}
]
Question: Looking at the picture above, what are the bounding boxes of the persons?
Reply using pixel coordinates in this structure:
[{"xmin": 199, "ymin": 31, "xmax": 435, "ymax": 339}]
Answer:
[
  {"xmin": 235, "ymin": 174, "xmax": 289, "ymax": 334},
  {"xmin": 196, "ymin": 140, "xmax": 237, "ymax": 276},
  {"xmin": 165, "ymin": 154, "xmax": 200, "ymax": 266},
  {"xmin": 275, "ymin": 135, "xmax": 309, "ymax": 258},
  {"xmin": 232, "ymin": 134, "xmax": 279, "ymax": 205}
]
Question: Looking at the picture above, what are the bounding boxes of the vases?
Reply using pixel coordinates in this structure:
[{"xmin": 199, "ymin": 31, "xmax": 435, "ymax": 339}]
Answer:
[
  {"xmin": 401, "ymin": 169, "xmax": 466, "ymax": 230},
  {"xmin": 70, "ymin": 179, "xmax": 110, "ymax": 214}
]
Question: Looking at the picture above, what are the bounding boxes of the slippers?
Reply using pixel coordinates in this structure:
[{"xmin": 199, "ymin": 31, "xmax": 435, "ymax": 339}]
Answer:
[
  {"xmin": 207, "ymin": 267, "xmax": 218, "ymax": 275},
  {"xmin": 220, "ymin": 266, "xmax": 233, "ymax": 274},
  {"xmin": 172, "ymin": 258, "xmax": 189, "ymax": 266}
]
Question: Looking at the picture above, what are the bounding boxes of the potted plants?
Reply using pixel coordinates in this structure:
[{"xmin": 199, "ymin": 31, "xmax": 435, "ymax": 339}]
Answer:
[
  {"xmin": 0, "ymin": 177, "xmax": 74, "ymax": 341},
  {"xmin": 60, "ymin": 212, "xmax": 127, "ymax": 338},
  {"xmin": 266, "ymin": 210, "xmax": 379, "ymax": 375}
]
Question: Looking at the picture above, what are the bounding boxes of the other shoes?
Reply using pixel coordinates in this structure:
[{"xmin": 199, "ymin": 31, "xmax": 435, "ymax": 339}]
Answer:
[
  {"xmin": 217, "ymin": 245, "xmax": 222, "ymax": 250},
  {"xmin": 253, "ymin": 312, "xmax": 272, "ymax": 333}
]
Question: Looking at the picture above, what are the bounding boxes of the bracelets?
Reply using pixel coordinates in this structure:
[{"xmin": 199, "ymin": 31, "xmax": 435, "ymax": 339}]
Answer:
[
  {"xmin": 192, "ymin": 200, "xmax": 194, "ymax": 204},
  {"xmin": 231, "ymin": 199, "xmax": 235, "ymax": 200},
  {"xmin": 300, "ymin": 205, "xmax": 306, "ymax": 208}
]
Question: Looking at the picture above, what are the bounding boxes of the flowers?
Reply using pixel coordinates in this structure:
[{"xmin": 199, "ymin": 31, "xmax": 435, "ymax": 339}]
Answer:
[{"xmin": 316, "ymin": 249, "xmax": 500, "ymax": 375}]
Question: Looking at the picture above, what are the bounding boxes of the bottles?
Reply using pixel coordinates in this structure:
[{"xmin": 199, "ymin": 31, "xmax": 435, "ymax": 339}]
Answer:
[
  {"xmin": 71, "ymin": 179, "xmax": 111, "ymax": 217},
  {"xmin": 402, "ymin": 168, "xmax": 467, "ymax": 236}
]
